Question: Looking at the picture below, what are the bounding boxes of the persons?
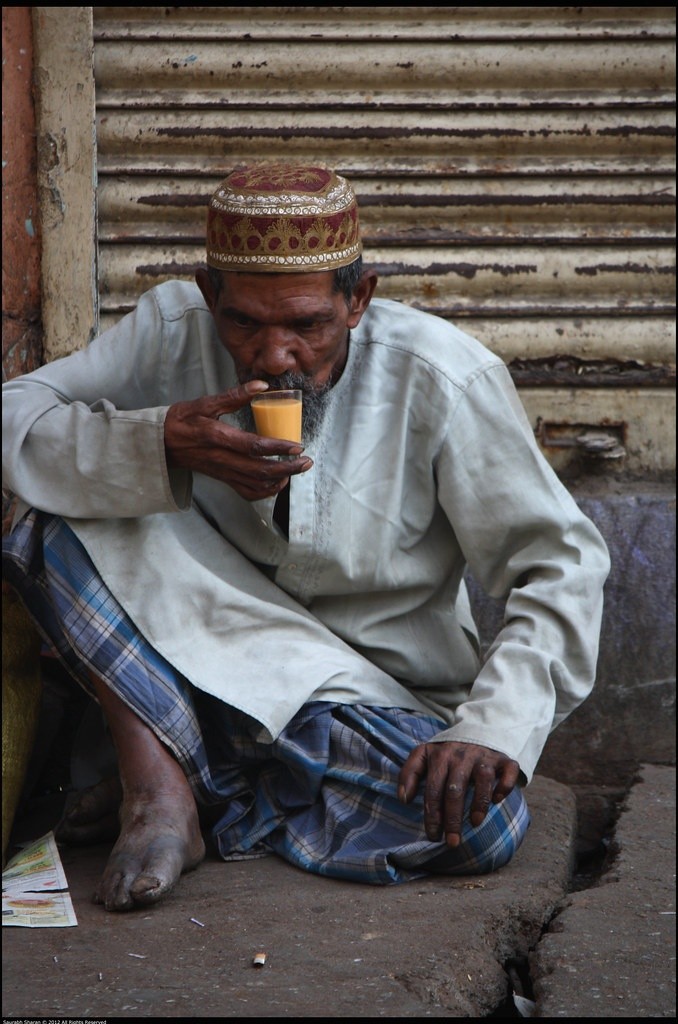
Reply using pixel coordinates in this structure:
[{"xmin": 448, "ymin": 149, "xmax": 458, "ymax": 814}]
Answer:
[{"xmin": 2, "ymin": 165, "xmax": 610, "ymax": 911}]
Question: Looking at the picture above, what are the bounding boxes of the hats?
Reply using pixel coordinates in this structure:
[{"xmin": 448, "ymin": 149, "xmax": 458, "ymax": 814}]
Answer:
[{"xmin": 206, "ymin": 164, "xmax": 363, "ymax": 270}]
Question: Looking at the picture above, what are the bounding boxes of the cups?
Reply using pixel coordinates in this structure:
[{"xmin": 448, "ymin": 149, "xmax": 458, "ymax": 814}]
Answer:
[{"xmin": 247, "ymin": 388, "xmax": 305, "ymax": 465}]
